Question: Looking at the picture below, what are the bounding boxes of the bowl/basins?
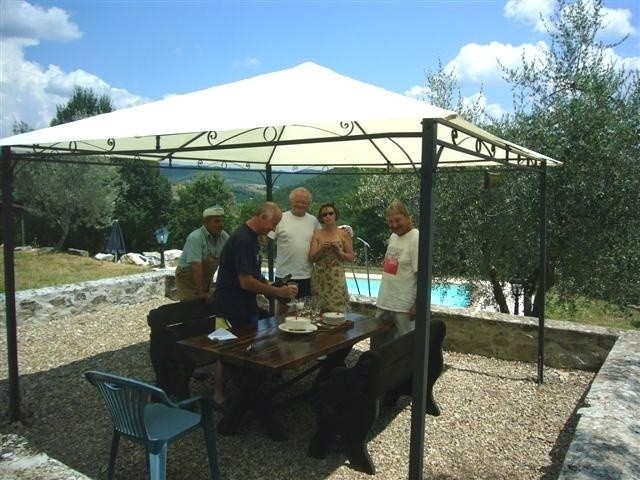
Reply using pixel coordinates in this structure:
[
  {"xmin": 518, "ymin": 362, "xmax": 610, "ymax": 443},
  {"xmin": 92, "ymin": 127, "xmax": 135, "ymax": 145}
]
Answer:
[{"xmin": 286, "ymin": 318, "xmax": 310, "ymax": 330}]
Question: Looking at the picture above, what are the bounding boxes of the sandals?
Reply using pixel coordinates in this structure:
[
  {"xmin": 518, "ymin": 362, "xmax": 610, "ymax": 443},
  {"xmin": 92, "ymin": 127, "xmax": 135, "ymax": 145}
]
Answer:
[{"xmin": 321, "ymin": 212, "xmax": 335, "ymax": 217}]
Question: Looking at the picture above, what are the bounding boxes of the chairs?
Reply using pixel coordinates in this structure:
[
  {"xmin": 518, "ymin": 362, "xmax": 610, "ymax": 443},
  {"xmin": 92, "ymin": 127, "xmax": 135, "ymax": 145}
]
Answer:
[{"xmin": 81, "ymin": 365, "xmax": 223, "ymax": 480}]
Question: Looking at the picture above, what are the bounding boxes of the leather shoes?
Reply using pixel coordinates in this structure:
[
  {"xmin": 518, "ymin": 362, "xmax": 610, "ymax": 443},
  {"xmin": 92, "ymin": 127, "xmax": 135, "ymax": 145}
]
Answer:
[{"xmin": 211, "ymin": 394, "xmax": 230, "ymax": 413}]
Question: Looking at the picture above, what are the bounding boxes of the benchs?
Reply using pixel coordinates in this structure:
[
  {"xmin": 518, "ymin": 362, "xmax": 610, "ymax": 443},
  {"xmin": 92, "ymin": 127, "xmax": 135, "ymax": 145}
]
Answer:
[
  {"xmin": 145, "ymin": 292, "xmax": 271, "ymax": 414},
  {"xmin": 303, "ymin": 317, "xmax": 449, "ymax": 475}
]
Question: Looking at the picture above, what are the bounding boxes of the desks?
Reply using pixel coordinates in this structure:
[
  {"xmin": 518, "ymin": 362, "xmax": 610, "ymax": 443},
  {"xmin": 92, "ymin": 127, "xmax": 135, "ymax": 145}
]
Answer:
[{"xmin": 172, "ymin": 301, "xmax": 398, "ymax": 446}]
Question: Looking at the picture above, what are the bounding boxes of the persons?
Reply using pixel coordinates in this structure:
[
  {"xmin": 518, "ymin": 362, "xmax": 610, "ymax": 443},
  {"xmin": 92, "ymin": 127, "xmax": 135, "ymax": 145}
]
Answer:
[
  {"xmin": 175, "ymin": 204, "xmax": 232, "ymax": 318},
  {"xmin": 369, "ymin": 201, "xmax": 419, "ymax": 358},
  {"xmin": 260, "ymin": 186, "xmax": 352, "ymax": 310},
  {"xmin": 213, "ymin": 201, "xmax": 297, "ymax": 414},
  {"xmin": 308, "ymin": 203, "xmax": 354, "ymax": 314}
]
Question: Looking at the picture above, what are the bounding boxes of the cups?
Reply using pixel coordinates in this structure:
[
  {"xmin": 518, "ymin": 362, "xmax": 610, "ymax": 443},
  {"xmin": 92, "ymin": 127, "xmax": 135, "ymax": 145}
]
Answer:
[
  {"xmin": 287, "ymin": 282, "xmax": 299, "ymax": 288},
  {"xmin": 295, "ymin": 296, "xmax": 320, "ymax": 321}
]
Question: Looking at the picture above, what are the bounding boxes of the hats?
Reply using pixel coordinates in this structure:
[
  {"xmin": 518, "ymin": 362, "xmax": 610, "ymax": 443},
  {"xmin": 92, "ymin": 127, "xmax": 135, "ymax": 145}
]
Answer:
[{"xmin": 202, "ymin": 206, "xmax": 225, "ymax": 218}]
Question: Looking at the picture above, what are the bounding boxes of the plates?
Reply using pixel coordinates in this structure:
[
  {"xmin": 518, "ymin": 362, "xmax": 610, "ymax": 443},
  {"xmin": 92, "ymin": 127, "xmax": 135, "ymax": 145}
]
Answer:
[
  {"xmin": 278, "ymin": 322, "xmax": 318, "ymax": 334},
  {"xmin": 322, "ymin": 312, "xmax": 345, "ymax": 319}
]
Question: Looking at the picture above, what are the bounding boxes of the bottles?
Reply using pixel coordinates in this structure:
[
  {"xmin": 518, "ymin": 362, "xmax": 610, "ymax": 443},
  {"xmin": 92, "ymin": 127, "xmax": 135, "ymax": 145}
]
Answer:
[{"xmin": 266, "ymin": 273, "xmax": 292, "ymax": 300}]
